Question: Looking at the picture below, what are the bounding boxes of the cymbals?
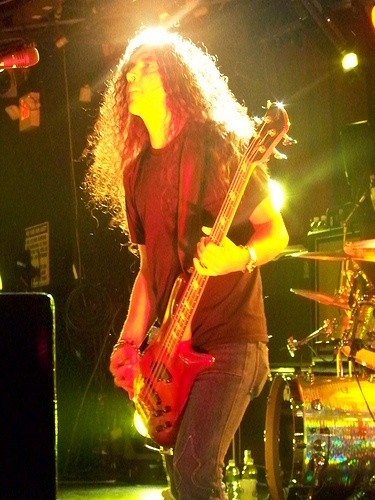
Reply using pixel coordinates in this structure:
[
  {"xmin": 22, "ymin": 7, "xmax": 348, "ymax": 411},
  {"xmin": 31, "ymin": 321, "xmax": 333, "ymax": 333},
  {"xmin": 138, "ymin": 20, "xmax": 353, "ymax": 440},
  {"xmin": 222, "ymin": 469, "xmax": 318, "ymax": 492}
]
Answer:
[
  {"xmin": 290, "ymin": 287, "xmax": 353, "ymax": 311},
  {"xmin": 343, "ymin": 238, "xmax": 375, "ymax": 262},
  {"xmin": 285, "ymin": 251, "xmax": 372, "ymax": 262}
]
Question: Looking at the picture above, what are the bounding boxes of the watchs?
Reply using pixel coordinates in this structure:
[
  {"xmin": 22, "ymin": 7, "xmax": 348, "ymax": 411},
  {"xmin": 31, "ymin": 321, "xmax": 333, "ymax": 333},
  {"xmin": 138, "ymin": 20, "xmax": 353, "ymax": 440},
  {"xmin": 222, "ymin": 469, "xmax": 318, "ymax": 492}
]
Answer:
[{"xmin": 240, "ymin": 245, "xmax": 256, "ymax": 273}]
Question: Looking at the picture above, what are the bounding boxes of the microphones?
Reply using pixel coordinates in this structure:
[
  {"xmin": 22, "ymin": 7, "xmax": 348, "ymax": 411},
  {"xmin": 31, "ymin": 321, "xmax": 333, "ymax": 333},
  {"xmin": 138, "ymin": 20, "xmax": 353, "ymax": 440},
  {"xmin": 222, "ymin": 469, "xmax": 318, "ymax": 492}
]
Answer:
[{"xmin": 0, "ymin": 47, "xmax": 39, "ymax": 68}]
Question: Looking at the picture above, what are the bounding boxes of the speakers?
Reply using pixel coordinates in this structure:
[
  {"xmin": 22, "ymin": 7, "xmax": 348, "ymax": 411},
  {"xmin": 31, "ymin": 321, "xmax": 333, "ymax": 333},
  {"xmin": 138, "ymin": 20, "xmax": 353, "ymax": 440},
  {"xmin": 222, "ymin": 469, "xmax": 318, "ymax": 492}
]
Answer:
[
  {"xmin": 0, "ymin": 292, "xmax": 60, "ymax": 500},
  {"xmin": 310, "ymin": 225, "xmax": 364, "ymax": 364}
]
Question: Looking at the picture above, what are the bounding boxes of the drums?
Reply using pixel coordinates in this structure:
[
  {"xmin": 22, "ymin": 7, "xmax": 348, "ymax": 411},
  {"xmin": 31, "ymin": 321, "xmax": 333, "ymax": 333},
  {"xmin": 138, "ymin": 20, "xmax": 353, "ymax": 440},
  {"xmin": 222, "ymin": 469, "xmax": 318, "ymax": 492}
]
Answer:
[
  {"xmin": 339, "ymin": 292, "xmax": 375, "ymax": 373},
  {"xmin": 264, "ymin": 371, "xmax": 375, "ymax": 500}
]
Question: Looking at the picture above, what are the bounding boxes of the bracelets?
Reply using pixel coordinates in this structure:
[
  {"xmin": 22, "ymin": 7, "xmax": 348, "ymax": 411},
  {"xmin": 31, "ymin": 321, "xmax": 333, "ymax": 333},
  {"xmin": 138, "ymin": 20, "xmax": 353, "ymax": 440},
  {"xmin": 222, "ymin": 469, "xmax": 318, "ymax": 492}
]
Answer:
[{"xmin": 113, "ymin": 339, "xmax": 140, "ymax": 353}]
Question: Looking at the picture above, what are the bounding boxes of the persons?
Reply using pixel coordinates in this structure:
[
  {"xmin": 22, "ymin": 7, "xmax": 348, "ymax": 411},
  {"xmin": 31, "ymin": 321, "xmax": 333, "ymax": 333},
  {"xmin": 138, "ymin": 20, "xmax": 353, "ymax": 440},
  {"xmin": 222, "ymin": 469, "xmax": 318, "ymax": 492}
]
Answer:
[{"xmin": 84, "ymin": 28, "xmax": 289, "ymax": 500}]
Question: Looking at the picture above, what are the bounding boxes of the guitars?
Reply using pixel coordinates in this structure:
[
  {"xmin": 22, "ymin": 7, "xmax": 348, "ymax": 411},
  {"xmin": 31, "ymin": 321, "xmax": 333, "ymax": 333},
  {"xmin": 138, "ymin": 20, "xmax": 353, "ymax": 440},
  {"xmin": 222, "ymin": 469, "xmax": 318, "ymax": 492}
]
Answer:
[{"xmin": 132, "ymin": 101, "xmax": 298, "ymax": 449}]
